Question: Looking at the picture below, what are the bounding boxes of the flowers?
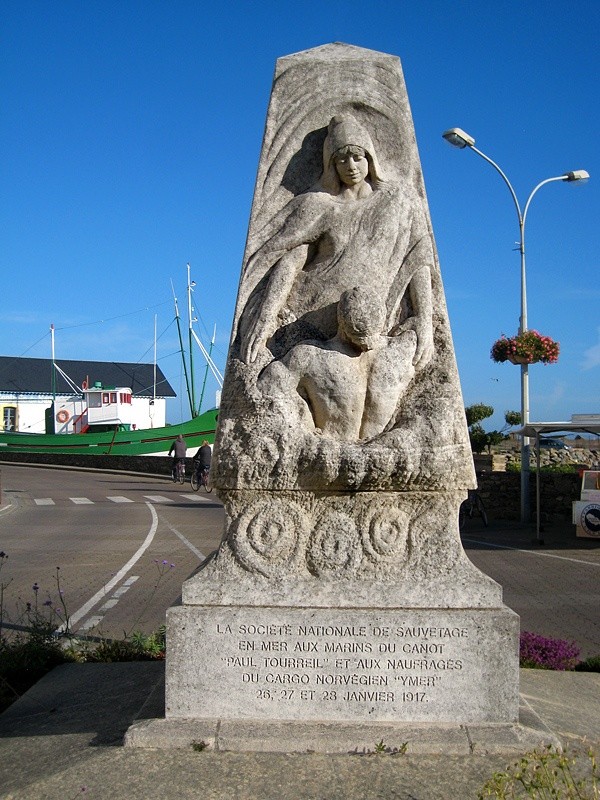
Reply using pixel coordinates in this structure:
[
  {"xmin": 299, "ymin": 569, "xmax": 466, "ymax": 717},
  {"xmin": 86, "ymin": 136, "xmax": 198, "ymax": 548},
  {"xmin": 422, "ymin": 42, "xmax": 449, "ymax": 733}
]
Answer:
[{"xmin": 489, "ymin": 328, "xmax": 559, "ymax": 367}]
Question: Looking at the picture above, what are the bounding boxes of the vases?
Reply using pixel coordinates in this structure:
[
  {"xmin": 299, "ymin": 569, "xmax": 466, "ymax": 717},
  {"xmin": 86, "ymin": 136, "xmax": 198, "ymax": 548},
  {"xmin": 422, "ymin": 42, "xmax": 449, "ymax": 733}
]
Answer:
[{"xmin": 506, "ymin": 350, "xmax": 540, "ymax": 366}]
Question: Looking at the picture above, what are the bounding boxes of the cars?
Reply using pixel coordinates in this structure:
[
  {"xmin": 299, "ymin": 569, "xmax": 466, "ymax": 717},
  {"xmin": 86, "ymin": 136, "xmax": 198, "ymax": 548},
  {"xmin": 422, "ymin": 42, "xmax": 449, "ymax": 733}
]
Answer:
[{"xmin": 534, "ymin": 439, "xmax": 569, "ymax": 450}]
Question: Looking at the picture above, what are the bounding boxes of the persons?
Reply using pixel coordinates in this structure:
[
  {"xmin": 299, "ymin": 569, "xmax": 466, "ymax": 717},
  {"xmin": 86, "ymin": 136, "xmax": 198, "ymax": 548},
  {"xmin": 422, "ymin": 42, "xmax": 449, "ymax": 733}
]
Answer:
[
  {"xmin": 191, "ymin": 440, "xmax": 213, "ymax": 487},
  {"xmin": 168, "ymin": 434, "xmax": 187, "ymax": 482},
  {"xmin": 240, "ymin": 114, "xmax": 435, "ymax": 447}
]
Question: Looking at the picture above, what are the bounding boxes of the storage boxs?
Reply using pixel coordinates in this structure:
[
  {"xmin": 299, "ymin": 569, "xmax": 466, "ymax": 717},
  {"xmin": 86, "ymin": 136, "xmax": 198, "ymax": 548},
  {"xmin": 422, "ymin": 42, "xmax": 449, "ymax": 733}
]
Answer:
[{"xmin": 475, "ymin": 452, "xmax": 507, "ymax": 472}]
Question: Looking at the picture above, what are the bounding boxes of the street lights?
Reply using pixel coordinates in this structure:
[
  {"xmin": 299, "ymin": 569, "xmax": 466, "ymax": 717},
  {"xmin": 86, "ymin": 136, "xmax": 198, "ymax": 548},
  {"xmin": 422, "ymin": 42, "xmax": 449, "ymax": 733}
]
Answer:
[
  {"xmin": 514, "ymin": 426, "xmax": 539, "ymax": 537},
  {"xmin": 441, "ymin": 126, "xmax": 591, "ymax": 525}
]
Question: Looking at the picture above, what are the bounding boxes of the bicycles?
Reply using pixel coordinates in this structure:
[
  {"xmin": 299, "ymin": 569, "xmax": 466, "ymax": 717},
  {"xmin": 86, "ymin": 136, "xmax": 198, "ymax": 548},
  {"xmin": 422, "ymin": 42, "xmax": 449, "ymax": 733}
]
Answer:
[
  {"xmin": 459, "ymin": 468, "xmax": 489, "ymax": 530},
  {"xmin": 189, "ymin": 457, "xmax": 213, "ymax": 493},
  {"xmin": 169, "ymin": 454, "xmax": 186, "ymax": 484}
]
